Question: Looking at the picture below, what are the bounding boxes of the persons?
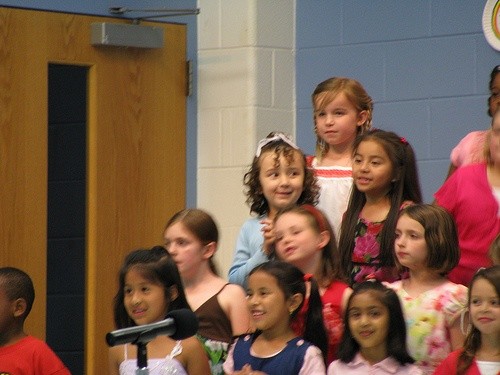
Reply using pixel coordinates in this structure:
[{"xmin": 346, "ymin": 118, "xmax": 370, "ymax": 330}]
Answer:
[
  {"xmin": 274, "ymin": 204, "xmax": 356, "ymax": 375},
  {"xmin": 338, "ymin": 129, "xmax": 424, "ymax": 290},
  {"xmin": 221, "ymin": 260, "xmax": 325, "ymax": 375},
  {"xmin": 227, "ymin": 130, "xmax": 320, "ymax": 286},
  {"xmin": 0, "ymin": 266, "xmax": 71, "ymax": 375},
  {"xmin": 377, "ymin": 204, "xmax": 472, "ymax": 375},
  {"xmin": 304, "ymin": 76, "xmax": 375, "ymax": 250},
  {"xmin": 162, "ymin": 208, "xmax": 255, "ymax": 375},
  {"xmin": 431, "ymin": 103, "xmax": 500, "ymax": 288},
  {"xmin": 108, "ymin": 246, "xmax": 211, "ymax": 375},
  {"xmin": 328, "ymin": 281, "xmax": 424, "ymax": 375},
  {"xmin": 443, "ymin": 65, "xmax": 500, "ymax": 185},
  {"xmin": 432, "ymin": 265, "xmax": 500, "ymax": 375}
]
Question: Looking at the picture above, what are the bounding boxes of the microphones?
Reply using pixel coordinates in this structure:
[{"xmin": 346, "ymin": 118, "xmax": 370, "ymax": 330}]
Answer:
[{"xmin": 107, "ymin": 310, "xmax": 199, "ymax": 347}]
